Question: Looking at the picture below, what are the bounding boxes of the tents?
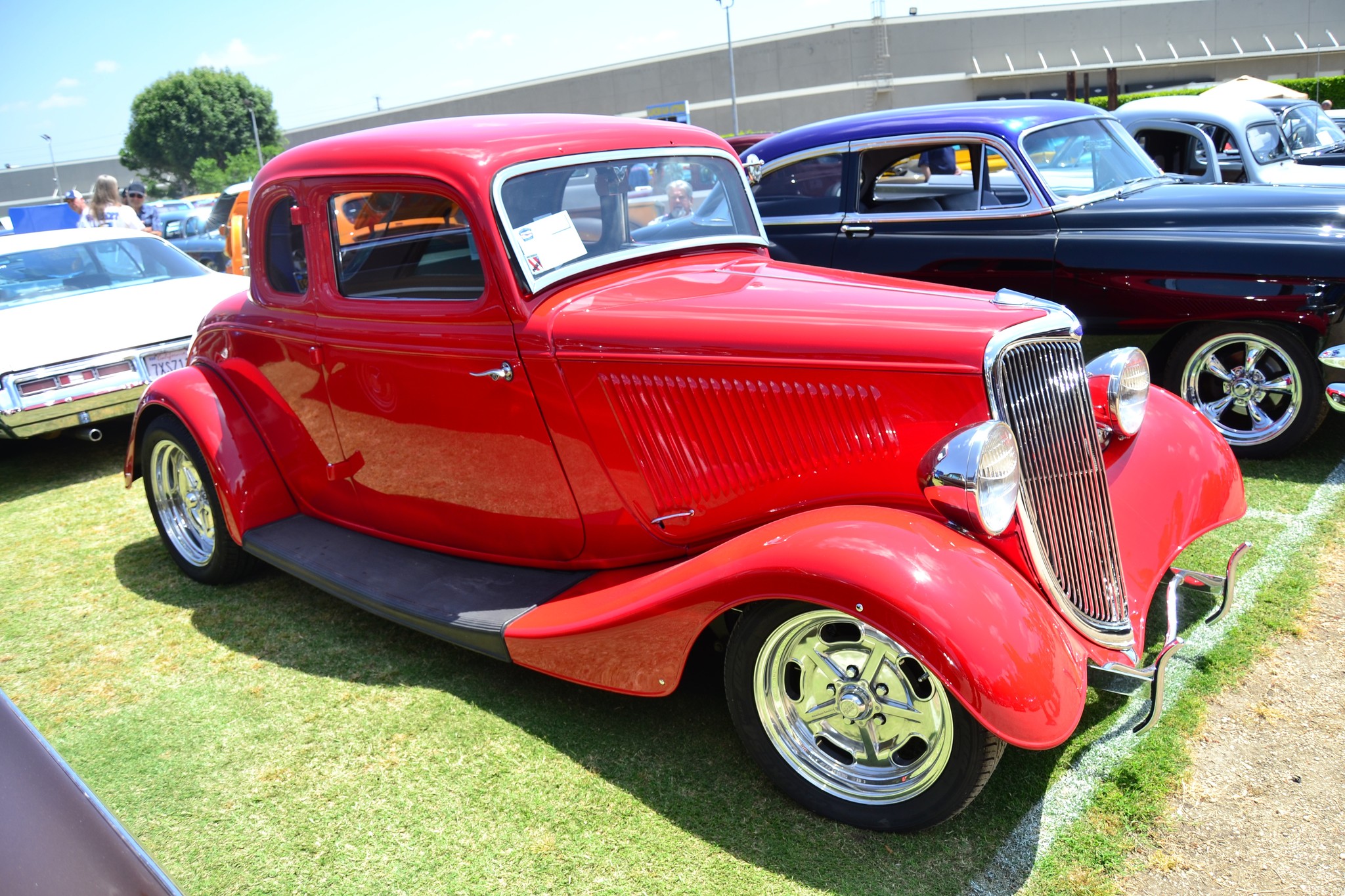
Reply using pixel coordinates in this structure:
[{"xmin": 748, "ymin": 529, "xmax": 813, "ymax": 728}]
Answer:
[{"xmin": 1199, "ymin": 74, "xmax": 1308, "ymax": 99}]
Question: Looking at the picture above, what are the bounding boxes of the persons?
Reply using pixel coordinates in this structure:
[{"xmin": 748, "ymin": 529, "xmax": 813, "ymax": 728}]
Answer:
[
  {"xmin": 647, "ymin": 180, "xmax": 694, "ymax": 225},
  {"xmin": 63, "ymin": 174, "xmax": 162, "ymax": 274},
  {"xmin": 1321, "ymin": 100, "xmax": 1332, "ymax": 110},
  {"xmin": 918, "ymin": 147, "xmax": 962, "ymax": 182},
  {"xmin": 628, "ymin": 163, "xmax": 654, "ymax": 191}
]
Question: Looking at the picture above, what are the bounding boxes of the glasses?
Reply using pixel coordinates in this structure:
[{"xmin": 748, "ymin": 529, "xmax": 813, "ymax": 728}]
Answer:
[{"xmin": 129, "ymin": 194, "xmax": 142, "ymax": 199}]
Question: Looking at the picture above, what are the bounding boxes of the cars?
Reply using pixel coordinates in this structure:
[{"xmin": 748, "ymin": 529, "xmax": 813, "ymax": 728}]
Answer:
[
  {"xmin": 629, "ymin": 98, "xmax": 1345, "ymax": 461},
  {"xmin": 121, "ymin": 112, "xmax": 1254, "ymax": 835},
  {"xmin": 134, "ymin": 162, "xmax": 711, "ymax": 283},
  {"xmin": 979, "ymin": 95, "xmax": 1345, "ymax": 194},
  {"xmin": 0, "ymin": 226, "xmax": 245, "ymax": 445}
]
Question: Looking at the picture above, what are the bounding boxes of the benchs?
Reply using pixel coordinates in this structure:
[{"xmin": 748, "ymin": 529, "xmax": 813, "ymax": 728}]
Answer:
[{"xmin": 894, "ymin": 190, "xmax": 1003, "ymax": 213}]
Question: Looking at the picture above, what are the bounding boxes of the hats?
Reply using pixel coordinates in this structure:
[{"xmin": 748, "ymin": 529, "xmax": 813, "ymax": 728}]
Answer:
[
  {"xmin": 128, "ymin": 182, "xmax": 146, "ymax": 196},
  {"xmin": 65, "ymin": 190, "xmax": 82, "ymax": 201}
]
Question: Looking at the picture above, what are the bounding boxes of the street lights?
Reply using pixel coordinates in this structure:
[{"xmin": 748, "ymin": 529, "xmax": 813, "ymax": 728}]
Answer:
[{"xmin": 38, "ymin": 132, "xmax": 63, "ymax": 203}]
